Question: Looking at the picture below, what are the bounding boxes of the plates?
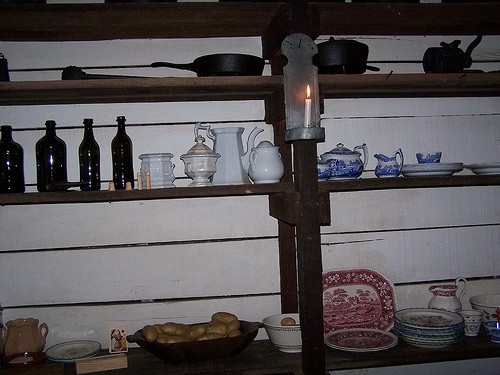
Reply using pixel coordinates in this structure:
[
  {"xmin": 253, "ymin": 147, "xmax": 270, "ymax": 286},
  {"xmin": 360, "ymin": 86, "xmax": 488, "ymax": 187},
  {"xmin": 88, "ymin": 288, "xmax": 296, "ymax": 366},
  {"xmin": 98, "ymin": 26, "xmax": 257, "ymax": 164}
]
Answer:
[
  {"xmin": 325, "ymin": 328, "xmax": 398, "ymax": 352},
  {"xmin": 400, "ymin": 162, "xmax": 464, "ymax": 176},
  {"xmin": 126, "ymin": 320, "xmax": 264, "ymax": 358},
  {"xmin": 464, "ymin": 162, "xmax": 500, "ymax": 175},
  {"xmin": 321, "ymin": 267, "xmax": 397, "ymax": 333},
  {"xmin": 45, "ymin": 340, "xmax": 102, "ymax": 362},
  {"xmin": 393, "ymin": 307, "xmax": 464, "ymax": 348}
]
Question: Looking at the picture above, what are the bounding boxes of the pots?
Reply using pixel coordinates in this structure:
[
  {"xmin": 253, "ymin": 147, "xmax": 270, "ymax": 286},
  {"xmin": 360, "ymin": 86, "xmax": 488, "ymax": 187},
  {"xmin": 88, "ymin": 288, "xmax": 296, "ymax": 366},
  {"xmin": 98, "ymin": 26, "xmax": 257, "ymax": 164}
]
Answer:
[
  {"xmin": 312, "ymin": 36, "xmax": 369, "ymax": 74},
  {"xmin": 151, "ymin": 53, "xmax": 264, "ymax": 77}
]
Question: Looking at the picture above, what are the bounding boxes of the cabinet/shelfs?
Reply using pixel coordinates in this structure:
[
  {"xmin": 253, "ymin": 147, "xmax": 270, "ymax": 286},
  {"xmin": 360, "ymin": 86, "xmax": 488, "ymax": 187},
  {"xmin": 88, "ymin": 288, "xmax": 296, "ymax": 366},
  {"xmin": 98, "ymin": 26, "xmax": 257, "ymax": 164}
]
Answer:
[{"xmin": 0, "ymin": 0, "xmax": 500, "ymax": 375}]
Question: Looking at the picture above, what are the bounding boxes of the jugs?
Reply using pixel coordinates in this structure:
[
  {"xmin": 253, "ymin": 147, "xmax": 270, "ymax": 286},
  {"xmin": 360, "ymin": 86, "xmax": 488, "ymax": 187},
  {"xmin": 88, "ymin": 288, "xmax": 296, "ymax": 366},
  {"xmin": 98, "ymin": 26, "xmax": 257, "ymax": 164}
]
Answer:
[
  {"xmin": 374, "ymin": 148, "xmax": 404, "ymax": 178},
  {"xmin": 135, "ymin": 153, "xmax": 176, "ymax": 190},
  {"xmin": 427, "ymin": 277, "xmax": 468, "ymax": 313}
]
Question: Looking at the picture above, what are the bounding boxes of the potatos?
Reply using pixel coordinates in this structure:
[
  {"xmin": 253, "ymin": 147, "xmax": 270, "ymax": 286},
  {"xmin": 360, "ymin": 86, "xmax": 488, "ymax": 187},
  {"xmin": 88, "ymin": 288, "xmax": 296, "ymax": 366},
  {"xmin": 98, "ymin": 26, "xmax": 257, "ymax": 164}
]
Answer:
[
  {"xmin": 281, "ymin": 317, "xmax": 296, "ymax": 326},
  {"xmin": 142, "ymin": 312, "xmax": 240, "ymax": 343}
]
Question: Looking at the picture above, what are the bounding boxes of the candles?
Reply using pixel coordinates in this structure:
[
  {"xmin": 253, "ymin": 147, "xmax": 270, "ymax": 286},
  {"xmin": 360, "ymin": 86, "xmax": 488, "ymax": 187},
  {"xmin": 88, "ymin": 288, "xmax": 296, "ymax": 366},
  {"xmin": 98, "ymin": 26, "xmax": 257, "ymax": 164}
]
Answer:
[{"xmin": 304, "ymin": 84, "xmax": 312, "ymax": 127}]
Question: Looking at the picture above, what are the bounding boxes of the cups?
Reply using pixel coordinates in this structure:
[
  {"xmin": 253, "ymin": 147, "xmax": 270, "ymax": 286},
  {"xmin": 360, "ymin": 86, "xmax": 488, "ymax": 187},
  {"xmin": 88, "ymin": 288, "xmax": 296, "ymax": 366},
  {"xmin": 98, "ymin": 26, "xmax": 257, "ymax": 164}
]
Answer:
[
  {"xmin": 460, "ymin": 311, "xmax": 481, "ymax": 336},
  {"xmin": 417, "ymin": 152, "xmax": 442, "ymax": 164},
  {"xmin": 180, "ymin": 135, "xmax": 221, "ymax": 187}
]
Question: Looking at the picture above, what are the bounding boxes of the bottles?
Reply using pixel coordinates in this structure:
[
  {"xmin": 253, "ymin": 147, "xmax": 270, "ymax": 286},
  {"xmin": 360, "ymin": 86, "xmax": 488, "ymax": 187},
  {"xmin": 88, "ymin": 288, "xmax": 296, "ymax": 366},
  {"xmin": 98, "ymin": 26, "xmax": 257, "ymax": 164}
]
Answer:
[
  {"xmin": 36, "ymin": 120, "xmax": 67, "ymax": 193},
  {"xmin": 79, "ymin": 118, "xmax": 101, "ymax": 191},
  {"xmin": 0, "ymin": 125, "xmax": 26, "ymax": 194},
  {"xmin": 111, "ymin": 116, "xmax": 134, "ymax": 190}
]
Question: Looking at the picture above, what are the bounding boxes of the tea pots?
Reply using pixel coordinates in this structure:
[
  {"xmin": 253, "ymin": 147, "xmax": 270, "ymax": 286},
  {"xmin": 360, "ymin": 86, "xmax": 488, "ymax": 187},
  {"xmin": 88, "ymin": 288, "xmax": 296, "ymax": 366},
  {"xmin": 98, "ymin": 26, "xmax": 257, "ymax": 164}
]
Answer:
[
  {"xmin": 194, "ymin": 123, "xmax": 264, "ymax": 186},
  {"xmin": 320, "ymin": 142, "xmax": 369, "ymax": 180},
  {"xmin": 248, "ymin": 140, "xmax": 284, "ymax": 184},
  {"xmin": 317, "ymin": 156, "xmax": 332, "ymax": 181},
  {"xmin": 2, "ymin": 318, "xmax": 49, "ymax": 368}
]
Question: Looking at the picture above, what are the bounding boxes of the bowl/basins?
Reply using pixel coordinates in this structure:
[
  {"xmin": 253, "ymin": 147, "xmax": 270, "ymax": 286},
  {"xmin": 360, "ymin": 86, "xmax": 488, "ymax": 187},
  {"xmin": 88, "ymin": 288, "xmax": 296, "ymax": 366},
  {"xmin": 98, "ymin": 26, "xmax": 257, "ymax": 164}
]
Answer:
[
  {"xmin": 469, "ymin": 294, "xmax": 500, "ymax": 344},
  {"xmin": 262, "ymin": 313, "xmax": 302, "ymax": 353}
]
